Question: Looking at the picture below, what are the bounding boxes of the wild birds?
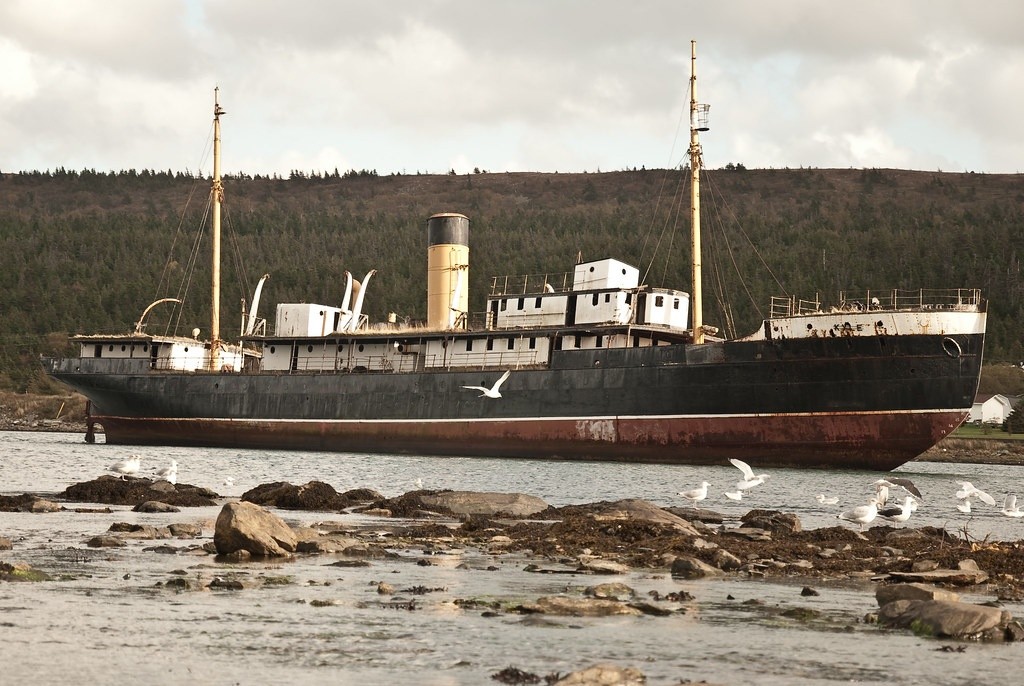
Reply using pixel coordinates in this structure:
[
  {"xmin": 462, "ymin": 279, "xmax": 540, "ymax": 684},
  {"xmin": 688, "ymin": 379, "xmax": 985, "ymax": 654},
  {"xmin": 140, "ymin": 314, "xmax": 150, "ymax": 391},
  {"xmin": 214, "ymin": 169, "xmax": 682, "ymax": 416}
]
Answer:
[
  {"xmin": 458, "ymin": 368, "xmax": 512, "ymax": 400},
  {"xmin": 676, "ymin": 458, "xmax": 1023, "ymax": 541},
  {"xmin": 222, "ymin": 476, "xmax": 237, "ymax": 486},
  {"xmin": 104, "ymin": 454, "xmax": 179, "ymax": 485}
]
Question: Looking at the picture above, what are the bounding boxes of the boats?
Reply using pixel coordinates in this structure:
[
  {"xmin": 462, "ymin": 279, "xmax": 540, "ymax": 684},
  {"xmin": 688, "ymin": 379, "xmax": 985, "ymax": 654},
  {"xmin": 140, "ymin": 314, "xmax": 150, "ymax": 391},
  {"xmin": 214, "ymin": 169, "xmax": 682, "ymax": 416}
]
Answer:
[{"xmin": 37, "ymin": 37, "xmax": 989, "ymax": 472}]
